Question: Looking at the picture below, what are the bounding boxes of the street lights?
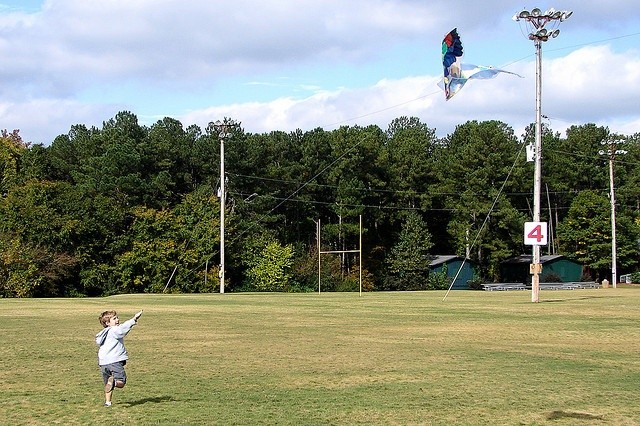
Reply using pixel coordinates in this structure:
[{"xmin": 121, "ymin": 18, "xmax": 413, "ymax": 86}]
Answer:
[{"xmin": 218, "ymin": 132, "xmax": 233, "ymax": 293}]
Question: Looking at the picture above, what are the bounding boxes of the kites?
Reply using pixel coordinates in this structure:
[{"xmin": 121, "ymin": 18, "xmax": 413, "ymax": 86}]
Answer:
[{"xmin": 435, "ymin": 26, "xmax": 526, "ymax": 102}]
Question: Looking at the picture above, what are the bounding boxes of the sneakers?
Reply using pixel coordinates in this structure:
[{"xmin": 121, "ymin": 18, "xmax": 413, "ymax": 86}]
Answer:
[
  {"xmin": 104, "ymin": 377, "xmax": 115, "ymax": 393},
  {"xmin": 105, "ymin": 402, "xmax": 112, "ymax": 407}
]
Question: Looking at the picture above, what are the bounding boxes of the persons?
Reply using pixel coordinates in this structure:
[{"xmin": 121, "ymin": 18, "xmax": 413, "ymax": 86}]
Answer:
[{"xmin": 95, "ymin": 309, "xmax": 144, "ymax": 407}]
